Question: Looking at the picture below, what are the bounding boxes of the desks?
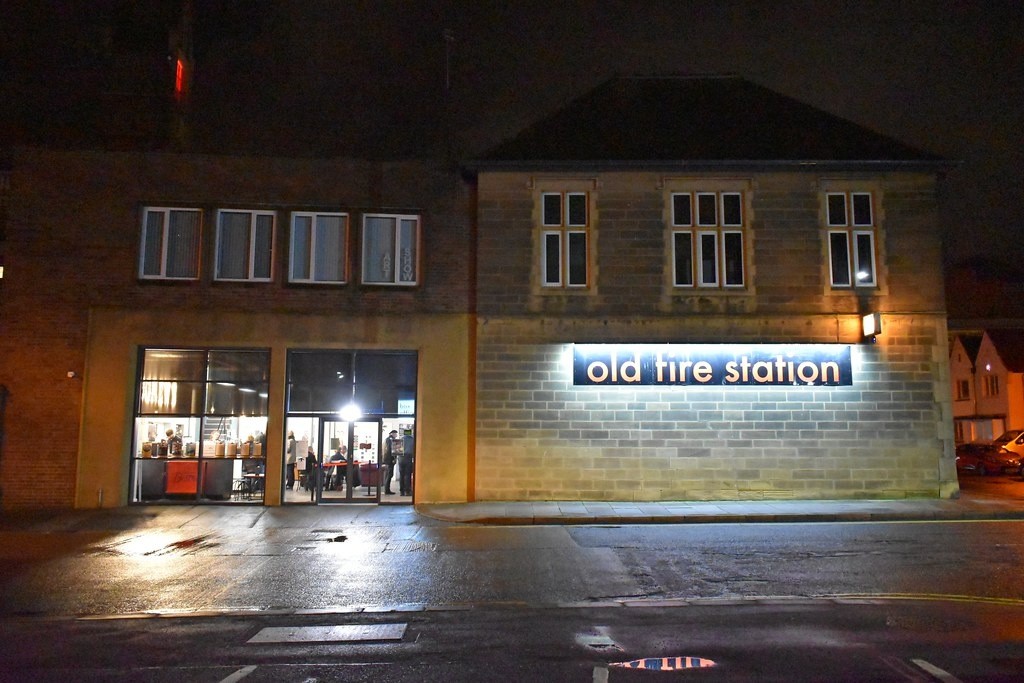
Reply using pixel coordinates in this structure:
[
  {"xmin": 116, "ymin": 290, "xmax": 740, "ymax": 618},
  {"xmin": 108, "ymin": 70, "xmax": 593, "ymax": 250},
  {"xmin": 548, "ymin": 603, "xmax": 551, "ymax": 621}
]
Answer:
[
  {"xmin": 243, "ymin": 474, "xmax": 265, "ymax": 500},
  {"xmin": 323, "ymin": 463, "xmax": 359, "ymax": 490}
]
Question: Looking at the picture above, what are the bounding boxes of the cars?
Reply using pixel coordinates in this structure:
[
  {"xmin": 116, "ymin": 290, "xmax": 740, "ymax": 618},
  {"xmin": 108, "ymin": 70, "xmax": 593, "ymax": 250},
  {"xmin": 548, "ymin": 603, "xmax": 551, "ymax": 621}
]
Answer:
[{"xmin": 955, "ymin": 442, "xmax": 1021, "ymax": 477}]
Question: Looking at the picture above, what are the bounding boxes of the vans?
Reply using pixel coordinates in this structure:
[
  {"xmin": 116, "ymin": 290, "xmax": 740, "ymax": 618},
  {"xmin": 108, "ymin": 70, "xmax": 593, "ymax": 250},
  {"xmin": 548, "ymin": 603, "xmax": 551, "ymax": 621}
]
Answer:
[{"xmin": 987, "ymin": 429, "xmax": 1024, "ymax": 462}]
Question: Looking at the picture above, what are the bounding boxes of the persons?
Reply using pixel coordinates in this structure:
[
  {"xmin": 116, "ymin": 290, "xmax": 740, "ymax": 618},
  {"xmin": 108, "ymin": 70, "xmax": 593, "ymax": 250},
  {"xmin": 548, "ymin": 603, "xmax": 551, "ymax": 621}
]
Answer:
[
  {"xmin": 167, "ymin": 429, "xmax": 182, "ymax": 454},
  {"xmin": 285, "ymin": 431, "xmax": 296, "ymax": 490},
  {"xmin": 383, "ymin": 430, "xmax": 414, "ymax": 496},
  {"xmin": 301, "ymin": 446, "xmax": 318, "ymax": 490},
  {"xmin": 326, "ymin": 446, "xmax": 349, "ymax": 491},
  {"xmin": 242, "ymin": 428, "xmax": 267, "ymax": 494}
]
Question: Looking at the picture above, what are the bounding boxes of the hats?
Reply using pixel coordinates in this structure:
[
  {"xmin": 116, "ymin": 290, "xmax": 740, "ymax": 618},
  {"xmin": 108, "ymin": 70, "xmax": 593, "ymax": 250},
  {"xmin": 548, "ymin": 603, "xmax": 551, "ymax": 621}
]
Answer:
[{"xmin": 388, "ymin": 429, "xmax": 398, "ymax": 434}]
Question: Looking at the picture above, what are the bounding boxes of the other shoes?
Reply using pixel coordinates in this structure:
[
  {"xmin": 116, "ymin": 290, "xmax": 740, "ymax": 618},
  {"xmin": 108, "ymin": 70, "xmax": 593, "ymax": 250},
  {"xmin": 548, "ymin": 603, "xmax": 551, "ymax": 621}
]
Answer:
[{"xmin": 386, "ymin": 491, "xmax": 396, "ymax": 494}]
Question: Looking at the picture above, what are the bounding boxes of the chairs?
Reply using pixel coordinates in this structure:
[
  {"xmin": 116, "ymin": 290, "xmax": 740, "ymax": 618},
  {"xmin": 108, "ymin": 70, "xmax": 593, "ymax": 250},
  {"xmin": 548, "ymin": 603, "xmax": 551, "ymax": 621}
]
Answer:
[{"xmin": 233, "ymin": 478, "xmax": 249, "ymax": 501}]
